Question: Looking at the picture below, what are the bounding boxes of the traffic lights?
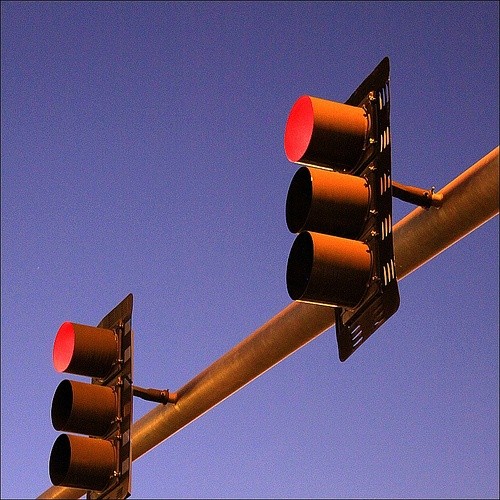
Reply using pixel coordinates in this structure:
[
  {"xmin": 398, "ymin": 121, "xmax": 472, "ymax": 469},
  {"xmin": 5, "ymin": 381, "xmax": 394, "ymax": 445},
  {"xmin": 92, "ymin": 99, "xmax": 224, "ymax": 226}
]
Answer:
[
  {"xmin": 284, "ymin": 56, "xmax": 400, "ymax": 362},
  {"xmin": 49, "ymin": 293, "xmax": 133, "ymax": 500}
]
